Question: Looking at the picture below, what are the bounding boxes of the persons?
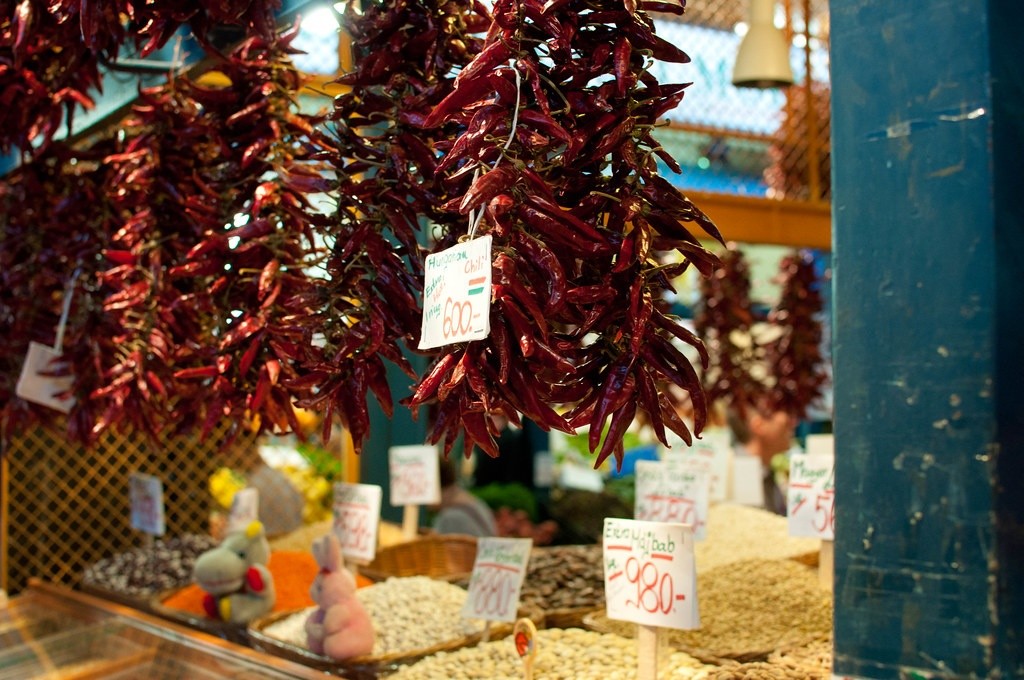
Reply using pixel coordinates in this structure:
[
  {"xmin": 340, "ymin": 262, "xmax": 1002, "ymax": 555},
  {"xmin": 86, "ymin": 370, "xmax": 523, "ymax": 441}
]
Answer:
[{"xmin": 424, "ymin": 451, "xmax": 500, "ymax": 539}]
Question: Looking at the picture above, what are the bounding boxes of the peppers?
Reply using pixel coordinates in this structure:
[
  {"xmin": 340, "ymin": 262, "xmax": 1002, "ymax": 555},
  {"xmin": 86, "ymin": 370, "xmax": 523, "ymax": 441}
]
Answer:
[{"xmin": 1, "ymin": 0, "xmax": 827, "ymax": 473}]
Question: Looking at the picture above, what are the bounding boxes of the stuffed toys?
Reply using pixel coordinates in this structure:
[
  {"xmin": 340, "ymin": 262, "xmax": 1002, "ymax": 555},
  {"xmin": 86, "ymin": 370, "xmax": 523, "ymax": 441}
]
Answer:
[
  {"xmin": 194, "ymin": 517, "xmax": 277, "ymax": 625},
  {"xmin": 302, "ymin": 535, "xmax": 375, "ymax": 660}
]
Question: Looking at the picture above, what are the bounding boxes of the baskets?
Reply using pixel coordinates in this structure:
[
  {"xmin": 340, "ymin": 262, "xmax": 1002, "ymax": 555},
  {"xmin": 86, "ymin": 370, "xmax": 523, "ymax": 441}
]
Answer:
[{"xmin": 80, "ymin": 529, "xmax": 821, "ymax": 679}]
